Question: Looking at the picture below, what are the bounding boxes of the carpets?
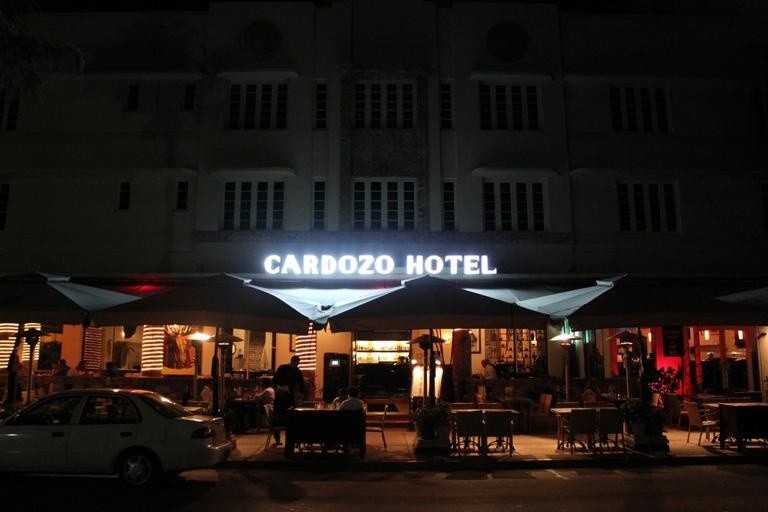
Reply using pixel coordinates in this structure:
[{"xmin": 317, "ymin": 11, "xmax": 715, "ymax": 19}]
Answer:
[{"xmin": 367, "ymin": 403, "xmax": 399, "ymax": 412}]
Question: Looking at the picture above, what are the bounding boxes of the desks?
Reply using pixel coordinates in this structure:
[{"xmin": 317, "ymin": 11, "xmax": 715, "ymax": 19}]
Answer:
[{"xmin": 32, "ymin": 366, "xmax": 266, "ymax": 436}]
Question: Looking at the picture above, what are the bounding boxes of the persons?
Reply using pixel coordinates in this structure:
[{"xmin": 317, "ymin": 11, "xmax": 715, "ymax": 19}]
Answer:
[
  {"xmin": 689, "ymin": 346, "xmax": 696, "ymax": 360},
  {"xmin": 704, "ymin": 352, "xmax": 715, "ymax": 361},
  {"xmin": 480, "ymin": 360, "xmax": 497, "ymax": 381},
  {"xmin": 584, "ymin": 376, "xmax": 617, "ymax": 401},
  {"xmin": 224, "ymin": 378, "xmax": 276, "ymax": 432},
  {"xmin": 50, "ymin": 360, "xmax": 119, "ymax": 391},
  {"xmin": 171, "ymin": 384, "xmax": 213, "ymax": 417},
  {"xmin": 339, "ymin": 388, "xmax": 365, "ymax": 411},
  {"xmin": 272, "ymin": 356, "xmax": 305, "ymax": 447},
  {"xmin": 331, "ymin": 388, "xmax": 349, "ymax": 411}
]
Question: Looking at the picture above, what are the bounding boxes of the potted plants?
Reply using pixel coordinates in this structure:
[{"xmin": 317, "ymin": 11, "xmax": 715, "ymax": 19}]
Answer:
[
  {"xmin": 618, "ymin": 396, "xmax": 649, "ymax": 439},
  {"xmin": 410, "ymin": 400, "xmax": 451, "ymax": 455}
]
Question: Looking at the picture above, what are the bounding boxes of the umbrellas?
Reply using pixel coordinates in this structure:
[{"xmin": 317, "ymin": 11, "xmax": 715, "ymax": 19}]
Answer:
[
  {"xmin": 519, "ymin": 274, "xmax": 728, "ymax": 369},
  {"xmin": 101, "ymin": 272, "xmax": 331, "ymax": 378},
  {"xmin": 0, "ymin": 271, "xmax": 142, "ymax": 364},
  {"xmin": 329, "ymin": 275, "xmax": 550, "ymax": 403},
  {"xmin": 717, "ymin": 286, "xmax": 767, "ymax": 326}
]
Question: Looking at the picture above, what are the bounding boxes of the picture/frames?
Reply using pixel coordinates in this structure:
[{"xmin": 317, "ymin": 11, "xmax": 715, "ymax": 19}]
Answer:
[{"xmin": 468, "ymin": 327, "xmax": 481, "ymax": 354}]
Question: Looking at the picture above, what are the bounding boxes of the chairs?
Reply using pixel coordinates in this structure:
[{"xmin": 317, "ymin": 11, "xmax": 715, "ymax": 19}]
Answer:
[
  {"xmin": 479, "ymin": 383, "xmax": 553, "ymax": 435},
  {"xmin": 672, "ymin": 396, "xmax": 767, "ymax": 452},
  {"xmin": 262, "ymin": 406, "xmax": 301, "ymax": 458},
  {"xmin": 365, "ymin": 401, "xmax": 390, "ymax": 453},
  {"xmin": 451, "ymin": 402, "xmax": 521, "ymax": 459},
  {"xmin": 549, "ymin": 400, "xmax": 628, "ymax": 453}
]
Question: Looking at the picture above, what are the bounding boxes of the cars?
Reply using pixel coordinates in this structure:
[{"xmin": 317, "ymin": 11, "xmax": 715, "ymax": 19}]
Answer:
[{"xmin": 0, "ymin": 387, "xmax": 233, "ymax": 489}]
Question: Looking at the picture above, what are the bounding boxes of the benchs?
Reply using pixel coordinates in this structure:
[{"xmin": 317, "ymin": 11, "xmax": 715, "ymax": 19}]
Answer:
[{"xmin": 286, "ymin": 409, "xmax": 366, "ymax": 457}]
